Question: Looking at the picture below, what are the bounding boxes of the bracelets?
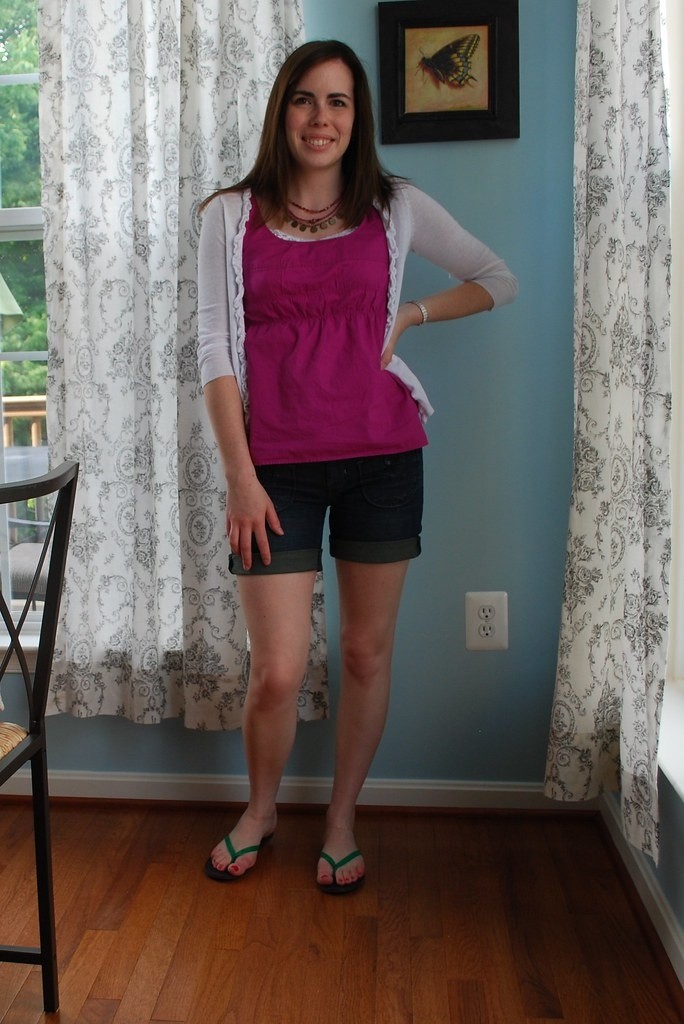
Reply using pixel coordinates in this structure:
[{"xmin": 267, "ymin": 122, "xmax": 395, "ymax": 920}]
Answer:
[{"xmin": 403, "ymin": 299, "xmax": 428, "ymax": 326}]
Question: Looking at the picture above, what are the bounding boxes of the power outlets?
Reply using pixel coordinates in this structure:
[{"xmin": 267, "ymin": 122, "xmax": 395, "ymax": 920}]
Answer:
[{"xmin": 465, "ymin": 591, "xmax": 508, "ymax": 650}]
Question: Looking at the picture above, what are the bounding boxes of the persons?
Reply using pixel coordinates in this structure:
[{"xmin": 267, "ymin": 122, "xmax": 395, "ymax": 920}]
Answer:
[{"xmin": 196, "ymin": 39, "xmax": 520, "ymax": 894}]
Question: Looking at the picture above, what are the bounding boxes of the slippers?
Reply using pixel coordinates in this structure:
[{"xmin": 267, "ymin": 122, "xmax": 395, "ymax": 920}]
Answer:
[
  {"xmin": 318, "ymin": 850, "xmax": 365, "ymax": 895},
  {"xmin": 205, "ymin": 832, "xmax": 275, "ymax": 882}
]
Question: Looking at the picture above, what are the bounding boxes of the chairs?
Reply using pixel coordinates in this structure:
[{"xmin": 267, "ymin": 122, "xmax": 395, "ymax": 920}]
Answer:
[{"xmin": 0, "ymin": 458, "xmax": 81, "ymax": 1012}]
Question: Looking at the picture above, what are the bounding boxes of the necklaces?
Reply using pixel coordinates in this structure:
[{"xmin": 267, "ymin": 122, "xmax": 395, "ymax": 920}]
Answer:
[{"xmin": 266, "ymin": 182, "xmax": 350, "ymax": 234}]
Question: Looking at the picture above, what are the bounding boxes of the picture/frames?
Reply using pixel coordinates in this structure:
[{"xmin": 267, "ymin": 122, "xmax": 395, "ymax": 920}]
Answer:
[{"xmin": 378, "ymin": 0, "xmax": 520, "ymax": 145}]
